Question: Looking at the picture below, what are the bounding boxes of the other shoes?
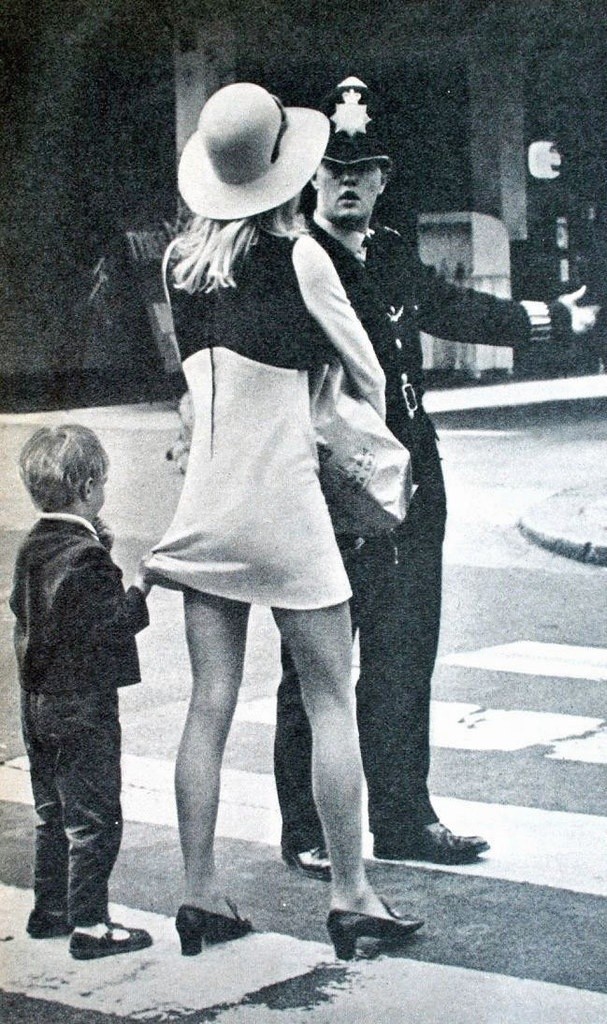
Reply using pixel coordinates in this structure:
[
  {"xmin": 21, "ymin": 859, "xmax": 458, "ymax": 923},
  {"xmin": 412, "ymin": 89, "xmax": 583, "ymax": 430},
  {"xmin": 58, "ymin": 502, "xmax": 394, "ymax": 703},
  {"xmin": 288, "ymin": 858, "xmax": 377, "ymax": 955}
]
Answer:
[
  {"xmin": 68, "ymin": 923, "xmax": 152, "ymax": 960},
  {"xmin": 24, "ymin": 909, "xmax": 71, "ymax": 940}
]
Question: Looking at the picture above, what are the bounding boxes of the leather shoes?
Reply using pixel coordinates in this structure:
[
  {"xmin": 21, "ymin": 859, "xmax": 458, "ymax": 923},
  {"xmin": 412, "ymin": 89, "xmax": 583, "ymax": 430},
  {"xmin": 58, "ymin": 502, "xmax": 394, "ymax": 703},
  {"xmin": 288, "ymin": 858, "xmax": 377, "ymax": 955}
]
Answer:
[
  {"xmin": 282, "ymin": 843, "xmax": 332, "ymax": 881},
  {"xmin": 373, "ymin": 822, "xmax": 490, "ymax": 864}
]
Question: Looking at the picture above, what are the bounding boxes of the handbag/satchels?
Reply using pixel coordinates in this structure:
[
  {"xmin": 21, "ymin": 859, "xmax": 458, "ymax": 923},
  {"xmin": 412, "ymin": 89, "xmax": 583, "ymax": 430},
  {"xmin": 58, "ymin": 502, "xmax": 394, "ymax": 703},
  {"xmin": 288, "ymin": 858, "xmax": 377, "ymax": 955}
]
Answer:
[{"xmin": 306, "ymin": 355, "xmax": 418, "ymax": 538}]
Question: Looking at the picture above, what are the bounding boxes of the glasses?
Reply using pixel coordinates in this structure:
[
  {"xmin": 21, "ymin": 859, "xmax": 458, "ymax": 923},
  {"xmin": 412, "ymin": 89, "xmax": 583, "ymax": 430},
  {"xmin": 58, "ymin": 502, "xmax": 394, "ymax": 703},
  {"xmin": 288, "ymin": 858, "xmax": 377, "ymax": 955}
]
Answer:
[{"xmin": 270, "ymin": 93, "xmax": 289, "ymax": 163}]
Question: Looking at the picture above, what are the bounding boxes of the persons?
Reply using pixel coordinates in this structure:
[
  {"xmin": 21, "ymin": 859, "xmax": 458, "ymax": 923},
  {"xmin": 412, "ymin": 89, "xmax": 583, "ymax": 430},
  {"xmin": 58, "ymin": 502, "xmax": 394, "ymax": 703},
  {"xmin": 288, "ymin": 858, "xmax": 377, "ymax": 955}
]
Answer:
[
  {"xmin": 269, "ymin": 79, "xmax": 597, "ymax": 878},
  {"xmin": 8, "ymin": 425, "xmax": 155, "ymax": 961},
  {"xmin": 145, "ymin": 83, "xmax": 424, "ymax": 958}
]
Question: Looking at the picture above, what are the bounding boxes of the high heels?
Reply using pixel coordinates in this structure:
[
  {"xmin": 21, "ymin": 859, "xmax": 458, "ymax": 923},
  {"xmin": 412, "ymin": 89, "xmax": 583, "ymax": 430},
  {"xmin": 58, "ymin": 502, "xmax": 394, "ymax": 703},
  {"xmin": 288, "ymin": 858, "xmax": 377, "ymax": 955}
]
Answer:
[
  {"xmin": 326, "ymin": 896, "xmax": 425, "ymax": 962},
  {"xmin": 175, "ymin": 898, "xmax": 252, "ymax": 955}
]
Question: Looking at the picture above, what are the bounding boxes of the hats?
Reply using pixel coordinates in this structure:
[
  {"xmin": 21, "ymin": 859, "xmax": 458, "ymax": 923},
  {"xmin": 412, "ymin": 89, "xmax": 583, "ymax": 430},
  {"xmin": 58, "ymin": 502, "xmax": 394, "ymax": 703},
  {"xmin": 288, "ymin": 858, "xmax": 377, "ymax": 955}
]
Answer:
[
  {"xmin": 315, "ymin": 76, "xmax": 395, "ymax": 171},
  {"xmin": 177, "ymin": 82, "xmax": 331, "ymax": 219}
]
